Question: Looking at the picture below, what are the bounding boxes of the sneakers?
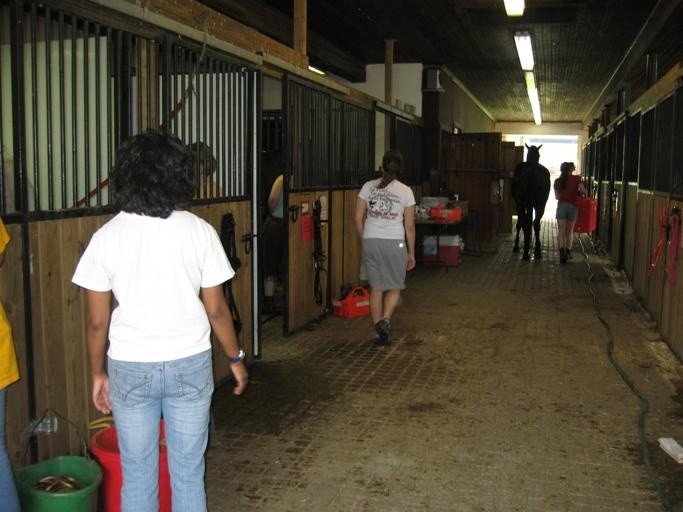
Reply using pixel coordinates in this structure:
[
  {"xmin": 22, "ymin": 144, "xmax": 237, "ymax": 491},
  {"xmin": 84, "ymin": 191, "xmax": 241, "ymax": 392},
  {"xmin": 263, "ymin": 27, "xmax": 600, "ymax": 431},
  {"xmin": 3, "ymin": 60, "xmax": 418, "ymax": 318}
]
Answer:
[
  {"xmin": 561, "ymin": 254, "xmax": 572, "ymax": 264},
  {"xmin": 375, "ymin": 318, "xmax": 392, "ymax": 345}
]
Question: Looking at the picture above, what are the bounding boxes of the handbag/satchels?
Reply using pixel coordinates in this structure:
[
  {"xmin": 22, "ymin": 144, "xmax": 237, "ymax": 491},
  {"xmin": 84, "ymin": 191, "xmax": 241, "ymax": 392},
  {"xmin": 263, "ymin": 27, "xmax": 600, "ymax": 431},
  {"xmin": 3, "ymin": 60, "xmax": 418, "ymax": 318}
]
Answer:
[
  {"xmin": 574, "ymin": 198, "xmax": 597, "ymax": 232},
  {"xmin": 332, "ymin": 286, "xmax": 371, "ymax": 318}
]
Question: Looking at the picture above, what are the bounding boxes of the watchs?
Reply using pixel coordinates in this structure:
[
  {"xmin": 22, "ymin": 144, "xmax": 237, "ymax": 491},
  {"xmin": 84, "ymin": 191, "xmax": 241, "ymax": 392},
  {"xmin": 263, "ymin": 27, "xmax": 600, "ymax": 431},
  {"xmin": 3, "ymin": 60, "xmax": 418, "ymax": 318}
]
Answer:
[{"xmin": 227, "ymin": 347, "xmax": 245, "ymax": 363}]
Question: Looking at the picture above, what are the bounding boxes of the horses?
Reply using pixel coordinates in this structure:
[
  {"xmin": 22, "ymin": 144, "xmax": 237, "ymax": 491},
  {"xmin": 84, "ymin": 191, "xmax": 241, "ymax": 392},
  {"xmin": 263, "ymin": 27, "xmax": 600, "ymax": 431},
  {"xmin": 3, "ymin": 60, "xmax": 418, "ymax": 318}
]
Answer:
[{"xmin": 512, "ymin": 142, "xmax": 551, "ymax": 262}]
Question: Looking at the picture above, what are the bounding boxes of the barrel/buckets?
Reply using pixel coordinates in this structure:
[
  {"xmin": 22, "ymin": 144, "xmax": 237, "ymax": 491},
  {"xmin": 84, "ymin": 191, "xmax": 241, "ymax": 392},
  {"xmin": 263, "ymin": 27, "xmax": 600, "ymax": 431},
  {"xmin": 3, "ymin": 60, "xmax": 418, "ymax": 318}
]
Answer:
[
  {"xmin": 12, "ymin": 408, "xmax": 104, "ymax": 511},
  {"xmin": 90, "ymin": 419, "xmax": 172, "ymax": 512}
]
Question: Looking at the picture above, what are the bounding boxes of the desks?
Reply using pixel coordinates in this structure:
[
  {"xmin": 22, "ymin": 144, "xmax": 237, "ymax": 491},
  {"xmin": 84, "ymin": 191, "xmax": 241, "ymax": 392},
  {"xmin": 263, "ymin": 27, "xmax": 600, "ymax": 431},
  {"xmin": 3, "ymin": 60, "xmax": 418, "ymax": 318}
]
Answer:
[{"xmin": 409, "ymin": 211, "xmax": 484, "ymax": 271}]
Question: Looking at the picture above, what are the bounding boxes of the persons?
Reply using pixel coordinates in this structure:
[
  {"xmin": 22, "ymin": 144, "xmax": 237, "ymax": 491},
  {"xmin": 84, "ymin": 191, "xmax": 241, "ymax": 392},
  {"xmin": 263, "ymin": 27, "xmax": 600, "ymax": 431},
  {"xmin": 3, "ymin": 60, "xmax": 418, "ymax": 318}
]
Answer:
[
  {"xmin": 553, "ymin": 162, "xmax": 587, "ymax": 264},
  {"xmin": 355, "ymin": 150, "xmax": 419, "ymax": 345},
  {"xmin": 183, "ymin": 141, "xmax": 223, "ymax": 201},
  {"xmin": 262, "ymin": 174, "xmax": 287, "ymax": 314},
  {"xmin": 69, "ymin": 131, "xmax": 249, "ymax": 511},
  {"xmin": 0, "ymin": 217, "xmax": 25, "ymax": 511}
]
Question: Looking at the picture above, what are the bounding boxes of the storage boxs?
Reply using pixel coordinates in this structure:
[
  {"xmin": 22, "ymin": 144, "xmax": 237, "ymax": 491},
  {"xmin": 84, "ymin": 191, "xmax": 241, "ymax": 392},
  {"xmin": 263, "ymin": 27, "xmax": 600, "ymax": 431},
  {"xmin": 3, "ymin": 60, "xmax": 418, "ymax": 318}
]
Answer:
[{"xmin": 423, "ymin": 245, "xmax": 463, "ymax": 267}]
